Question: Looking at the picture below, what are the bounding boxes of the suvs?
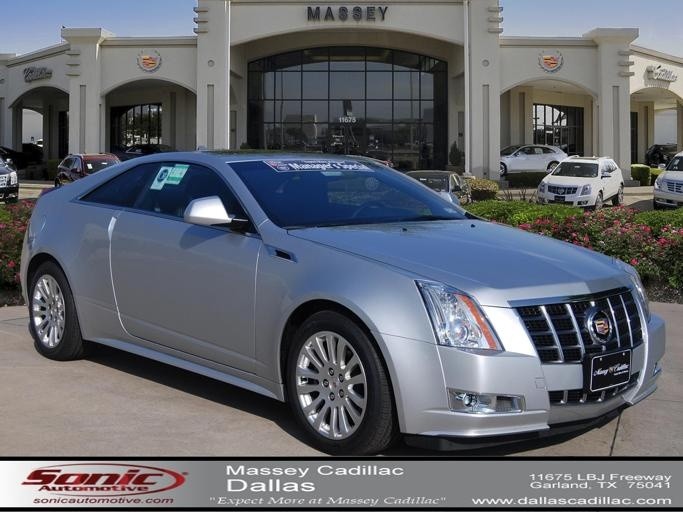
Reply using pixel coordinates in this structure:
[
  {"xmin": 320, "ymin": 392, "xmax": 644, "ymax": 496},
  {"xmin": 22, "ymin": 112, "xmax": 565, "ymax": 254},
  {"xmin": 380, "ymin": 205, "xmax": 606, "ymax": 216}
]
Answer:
[
  {"xmin": 644, "ymin": 143, "xmax": 676, "ymax": 170},
  {"xmin": 535, "ymin": 154, "xmax": 624, "ymax": 211}
]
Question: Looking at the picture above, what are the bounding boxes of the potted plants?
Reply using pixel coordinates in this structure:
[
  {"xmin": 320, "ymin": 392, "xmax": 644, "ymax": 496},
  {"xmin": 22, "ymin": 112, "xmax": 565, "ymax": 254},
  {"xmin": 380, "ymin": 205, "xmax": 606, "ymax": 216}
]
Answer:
[{"xmin": 446, "ymin": 140, "xmax": 464, "ymax": 175}]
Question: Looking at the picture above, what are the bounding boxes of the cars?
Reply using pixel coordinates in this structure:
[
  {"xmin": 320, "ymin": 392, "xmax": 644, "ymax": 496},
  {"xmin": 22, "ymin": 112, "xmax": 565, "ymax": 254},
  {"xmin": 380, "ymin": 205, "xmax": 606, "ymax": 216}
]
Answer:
[
  {"xmin": 19, "ymin": 149, "xmax": 665, "ymax": 456},
  {"xmin": 54, "ymin": 152, "xmax": 121, "ymax": 189},
  {"xmin": 309, "ymin": 143, "xmax": 438, "ymax": 170},
  {"xmin": 499, "ymin": 144, "xmax": 568, "ymax": 177},
  {"xmin": 653, "ymin": 150, "xmax": 683, "ymax": 211},
  {"xmin": 0, "ymin": 158, "xmax": 18, "ymax": 204},
  {"xmin": 21, "ymin": 138, "xmax": 43, "ymax": 162},
  {"xmin": 111, "ymin": 142, "xmax": 181, "ymax": 162},
  {"xmin": 0, "ymin": 146, "xmax": 28, "ymax": 175},
  {"xmin": 384, "ymin": 170, "xmax": 471, "ymax": 209},
  {"xmin": 266, "ymin": 154, "xmax": 396, "ymax": 195}
]
